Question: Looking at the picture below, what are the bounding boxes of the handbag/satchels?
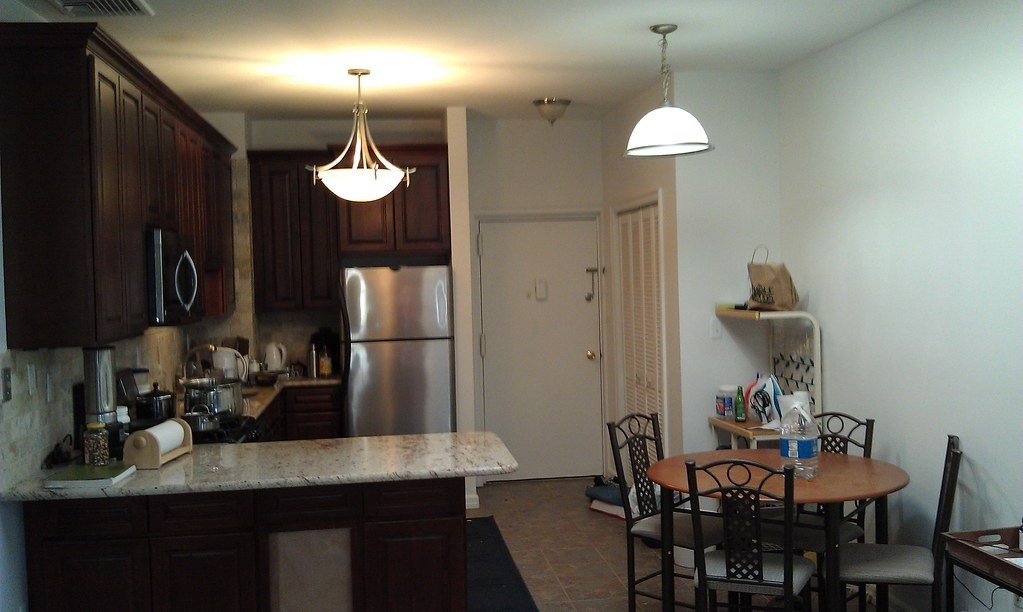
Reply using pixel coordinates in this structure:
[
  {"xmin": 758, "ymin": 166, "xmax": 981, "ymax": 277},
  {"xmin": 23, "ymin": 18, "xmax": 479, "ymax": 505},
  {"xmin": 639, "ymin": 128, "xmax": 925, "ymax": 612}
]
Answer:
[{"xmin": 746, "ymin": 245, "xmax": 799, "ymax": 311}]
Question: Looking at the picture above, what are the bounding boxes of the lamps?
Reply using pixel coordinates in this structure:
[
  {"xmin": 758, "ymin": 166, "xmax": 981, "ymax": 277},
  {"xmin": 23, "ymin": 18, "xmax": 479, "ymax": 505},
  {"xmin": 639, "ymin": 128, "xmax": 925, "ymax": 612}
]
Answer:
[
  {"xmin": 306, "ymin": 69, "xmax": 415, "ymax": 202},
  {"xmin": 625, "ymin": 24, "xmax": 710, "ymax": 156},
  {"xmin": 532, "ymin": 99, "xmax": 571, "ymax": 125}
]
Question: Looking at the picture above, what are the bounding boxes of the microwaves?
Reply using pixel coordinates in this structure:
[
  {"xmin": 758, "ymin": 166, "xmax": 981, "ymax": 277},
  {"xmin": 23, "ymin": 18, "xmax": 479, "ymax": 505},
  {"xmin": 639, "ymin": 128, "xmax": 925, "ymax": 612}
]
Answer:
[{"xmin": 146, "ymin": 223, "xmax": 199, "ymax": 326}]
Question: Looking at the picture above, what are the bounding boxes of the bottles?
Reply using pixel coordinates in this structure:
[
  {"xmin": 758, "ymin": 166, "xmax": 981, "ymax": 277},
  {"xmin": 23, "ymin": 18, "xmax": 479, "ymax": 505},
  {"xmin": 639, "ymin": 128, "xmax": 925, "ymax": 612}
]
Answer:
[
  {"xmin": 117, "ymin": 406, "xmax": 131, "ymax": 453},
  {"xmin": 714, "ymin": 386, "xmax": 737, "ymax": 420},
  {"xmin": 735, "ymin": 386, "xmax": 746, "ymax": 423},
  {"xmin": 84, "ymin": 423, "xmax": 109, "ymax": 466},
  {"xmin": 319, "ymin": 345, "xmax": 332, "ymax": 379},
  {"xmin": 249, "ymin": 360, "xmax": 259, "ymax": 385},
  {"xmin": 290, "ymin": 364, "xmax": 296, "ymax": 379},
  {"xmin": 780, "ymin": 401, "xmax": 819, "ymax": 481}
]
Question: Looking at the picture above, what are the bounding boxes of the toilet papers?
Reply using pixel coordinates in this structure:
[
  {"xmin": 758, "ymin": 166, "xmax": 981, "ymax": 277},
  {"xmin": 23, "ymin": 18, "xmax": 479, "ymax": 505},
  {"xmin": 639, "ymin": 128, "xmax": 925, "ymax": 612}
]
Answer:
[
  {"xmin": 141, "ymin": 420, "xmax": 184, "ymax": 455},
  {"xmin": 776, "ymin": 391, "xmax": 810, "ymax": 431}
]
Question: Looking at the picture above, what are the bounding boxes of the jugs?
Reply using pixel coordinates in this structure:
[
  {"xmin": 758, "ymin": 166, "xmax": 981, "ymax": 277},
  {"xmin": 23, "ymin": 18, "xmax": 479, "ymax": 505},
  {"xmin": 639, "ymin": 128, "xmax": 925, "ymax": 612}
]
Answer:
[{"xmin": 307, "ymin": 343, "xmax": 318, "ymax": 379}]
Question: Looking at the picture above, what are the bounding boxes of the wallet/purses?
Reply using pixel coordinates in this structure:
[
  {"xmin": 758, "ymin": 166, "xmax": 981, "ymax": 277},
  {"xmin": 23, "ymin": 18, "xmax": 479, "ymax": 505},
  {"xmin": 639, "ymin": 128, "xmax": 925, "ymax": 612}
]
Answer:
[{"xmin": 43, "ymin": 433, "xmax": 72, "ymax": 470}]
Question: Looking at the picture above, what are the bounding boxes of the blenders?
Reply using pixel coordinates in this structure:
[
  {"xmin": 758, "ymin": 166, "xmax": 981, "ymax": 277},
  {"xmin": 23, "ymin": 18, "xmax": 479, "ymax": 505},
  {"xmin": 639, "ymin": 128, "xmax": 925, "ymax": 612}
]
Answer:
[{"xmin": 82, "ymin": 346, "xmax": 125, "ymax": 462}]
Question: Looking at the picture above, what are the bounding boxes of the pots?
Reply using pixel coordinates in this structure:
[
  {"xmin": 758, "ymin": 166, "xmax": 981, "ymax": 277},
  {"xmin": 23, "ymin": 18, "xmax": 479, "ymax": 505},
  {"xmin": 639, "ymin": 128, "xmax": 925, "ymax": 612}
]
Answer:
[
  {"xmin": 181, "ymin": 403, "xmax": 232, "ymax": 433},
  {"xmin": 135, "ymin": 382, "xmax": 174, "ymax": 426},
  {"xmin": 257, "ymin": 371, "xmax": 278, "ymax": 388},
  {"xmin": 177, "ymin": 379, "xmax": 244, "ymax": 423}
]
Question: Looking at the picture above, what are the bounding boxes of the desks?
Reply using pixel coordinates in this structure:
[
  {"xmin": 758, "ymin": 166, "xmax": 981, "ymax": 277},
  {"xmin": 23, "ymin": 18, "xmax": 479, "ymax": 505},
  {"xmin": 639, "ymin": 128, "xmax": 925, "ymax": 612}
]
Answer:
[
  {"xmin": 647, "ymin": 449, "xmax": 910, "ymax": 612},
  {"xmin": 945, "ymin": 550, "xmax": 1023, "ymax": 612}
]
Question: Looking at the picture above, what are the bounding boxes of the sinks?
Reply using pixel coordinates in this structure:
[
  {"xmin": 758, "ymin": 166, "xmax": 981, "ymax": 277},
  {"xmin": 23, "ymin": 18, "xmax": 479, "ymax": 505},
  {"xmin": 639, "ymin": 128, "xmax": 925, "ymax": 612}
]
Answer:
[{"xmin": 242, "ymin": 391, "xmax": 258, "ymax": 400}]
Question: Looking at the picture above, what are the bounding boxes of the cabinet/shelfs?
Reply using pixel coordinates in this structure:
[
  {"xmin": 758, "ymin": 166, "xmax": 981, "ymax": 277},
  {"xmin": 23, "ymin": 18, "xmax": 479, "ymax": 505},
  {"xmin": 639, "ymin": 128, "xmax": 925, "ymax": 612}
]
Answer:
[
  {"xmin": 709, "ymin": 305, "xmax": 823, "ymax": 553},
  {"xmin": 0, "ymin": 21, "xmax": 142, "ymax": 349},
  {"xmin": 180, "ymin": 99, "xmax": 238, "ymax": 318},
  {"xmin": 23, "ymin": 477, "xmax": 469, "ymax": 612},
  {"xmin": 141, "ymin": 65, "xmax": 180, "ymax": 225},
  {"xmin": 247, "ymin": 150, "xmax": 340, "ymax": 312},
  {"xmin": 257, "ymin": 387, "xmax": 285, "ymax": 441},
  {"xmin": 285, "ymin": 386, "xmax": 345, "ymax": 439},
  {"xmin": 327, "ymin": 142, "xmax": 450, "ymax": 256}
]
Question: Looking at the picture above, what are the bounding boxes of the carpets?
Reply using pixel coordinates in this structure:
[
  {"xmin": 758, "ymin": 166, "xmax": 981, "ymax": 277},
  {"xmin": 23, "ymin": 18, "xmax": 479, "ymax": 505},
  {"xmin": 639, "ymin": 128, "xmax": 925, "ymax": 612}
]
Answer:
[{"xmin": 466, "ymin": 515, "xmax": 540, "ymax": 612}]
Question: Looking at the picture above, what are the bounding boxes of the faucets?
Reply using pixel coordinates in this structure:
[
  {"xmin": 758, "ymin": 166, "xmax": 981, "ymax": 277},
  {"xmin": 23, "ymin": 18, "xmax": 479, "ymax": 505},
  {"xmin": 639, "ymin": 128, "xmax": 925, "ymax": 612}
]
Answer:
[{"xmin": 173, "ymin": 344, "xmax": 218, "ymax": 392}]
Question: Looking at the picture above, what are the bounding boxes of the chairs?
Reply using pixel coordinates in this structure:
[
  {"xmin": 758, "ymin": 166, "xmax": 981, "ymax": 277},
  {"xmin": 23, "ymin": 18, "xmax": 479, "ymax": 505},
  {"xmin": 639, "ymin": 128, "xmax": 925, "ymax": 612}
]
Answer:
[{"xmin": 606, "ymin": 411, "xmax": 963, "ymax": 612}]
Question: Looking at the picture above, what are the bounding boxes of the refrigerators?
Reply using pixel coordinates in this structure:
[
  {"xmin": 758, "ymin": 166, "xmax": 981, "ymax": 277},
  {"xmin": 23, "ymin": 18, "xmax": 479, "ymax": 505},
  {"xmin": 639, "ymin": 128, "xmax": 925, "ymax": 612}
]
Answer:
[{"xmin": 335, "ymin": 265, "xmax": 456, "ymax": 437}]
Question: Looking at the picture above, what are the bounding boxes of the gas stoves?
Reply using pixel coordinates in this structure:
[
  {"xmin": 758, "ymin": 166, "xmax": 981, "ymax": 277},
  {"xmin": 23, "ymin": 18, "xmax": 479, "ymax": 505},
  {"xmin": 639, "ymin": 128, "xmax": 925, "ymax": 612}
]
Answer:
[{"xmin": 179, "ymin": 415, "xmax": 258, "ymax": 443}]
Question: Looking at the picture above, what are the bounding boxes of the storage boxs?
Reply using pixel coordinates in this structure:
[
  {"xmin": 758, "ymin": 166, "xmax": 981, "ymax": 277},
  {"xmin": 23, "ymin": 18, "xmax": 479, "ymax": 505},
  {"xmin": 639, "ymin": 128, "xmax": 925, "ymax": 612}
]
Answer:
[{"xmin": 939, "ymin": 525, "xmax": 1023, "ymax": 590}]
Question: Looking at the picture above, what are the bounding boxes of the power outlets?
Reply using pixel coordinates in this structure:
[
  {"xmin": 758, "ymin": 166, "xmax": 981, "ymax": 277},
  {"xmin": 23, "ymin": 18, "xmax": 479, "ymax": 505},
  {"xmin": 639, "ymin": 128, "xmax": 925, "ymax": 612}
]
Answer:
[
  {"xmin": 1014, "ymin": 593, "xmax": 1023, "ymax": 610},
  {"xmin": 2, "ymin": 368, "xmax": 12, "ymax": 401}
]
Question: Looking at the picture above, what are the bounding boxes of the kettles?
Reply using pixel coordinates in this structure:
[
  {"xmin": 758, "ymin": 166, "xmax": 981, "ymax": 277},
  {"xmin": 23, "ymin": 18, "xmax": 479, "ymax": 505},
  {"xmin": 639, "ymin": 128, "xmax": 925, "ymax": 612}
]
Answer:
[
  {"xmin": 262, "ymin": 342, "xmax": 287, "ymax": 372},
  {"xmin": 212, "ymin": 347, "xmax": 247, "ymax": 380}
]
